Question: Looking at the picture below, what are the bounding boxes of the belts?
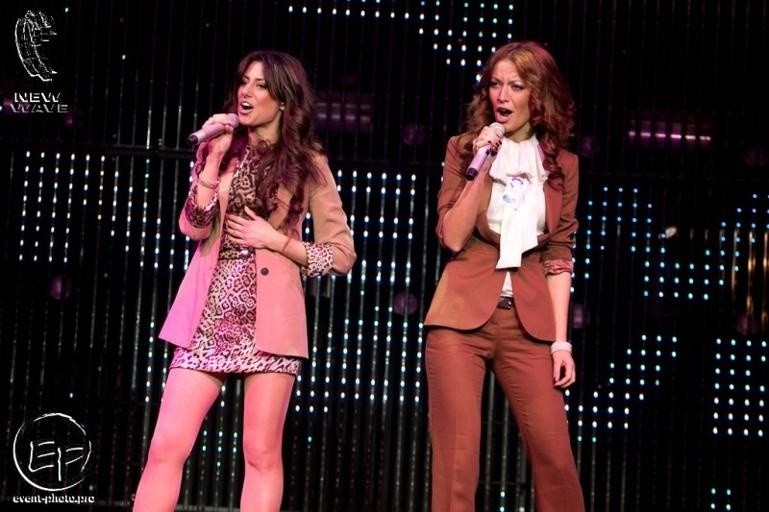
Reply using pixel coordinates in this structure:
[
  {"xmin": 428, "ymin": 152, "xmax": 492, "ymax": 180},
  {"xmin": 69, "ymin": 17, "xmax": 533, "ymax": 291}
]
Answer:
[{"xmin": 498, "ymin": 292, "xmax": 515, "ymax": 312}]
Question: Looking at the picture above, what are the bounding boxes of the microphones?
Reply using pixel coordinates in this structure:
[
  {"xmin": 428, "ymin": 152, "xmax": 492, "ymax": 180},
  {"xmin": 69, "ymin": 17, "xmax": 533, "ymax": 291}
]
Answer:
[
  {"xmin": 186, "ymin": 112, "xmax": 239, "ymax": 145},
  {"xmin": 466, "ymin": 123, "xmax": 506, "ymax": 180}
]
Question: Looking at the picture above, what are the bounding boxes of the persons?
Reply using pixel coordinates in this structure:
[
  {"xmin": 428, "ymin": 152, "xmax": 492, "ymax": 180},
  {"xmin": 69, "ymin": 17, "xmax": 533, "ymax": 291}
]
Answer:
[
  {"xmin": 129, "ymin": 47, "xmax": 359, "ymax": 512},
  {"xmin": 421, "ymin": 41, "xmax": 588, "ymax": 511}
]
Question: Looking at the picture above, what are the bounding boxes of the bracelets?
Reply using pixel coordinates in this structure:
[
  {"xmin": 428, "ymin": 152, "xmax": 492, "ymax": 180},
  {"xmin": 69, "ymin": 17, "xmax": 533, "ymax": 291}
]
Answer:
[
  {"xmin": 196, "ymin": 174, "xmax": 220, "ymax": 189},
  {"xmin": 281, "ymin": 237, "xmax": 293, "ymax": 253},
  {"xmin": 550, "ymin": 341, "xmax": 572, "ymax": 354}
]
